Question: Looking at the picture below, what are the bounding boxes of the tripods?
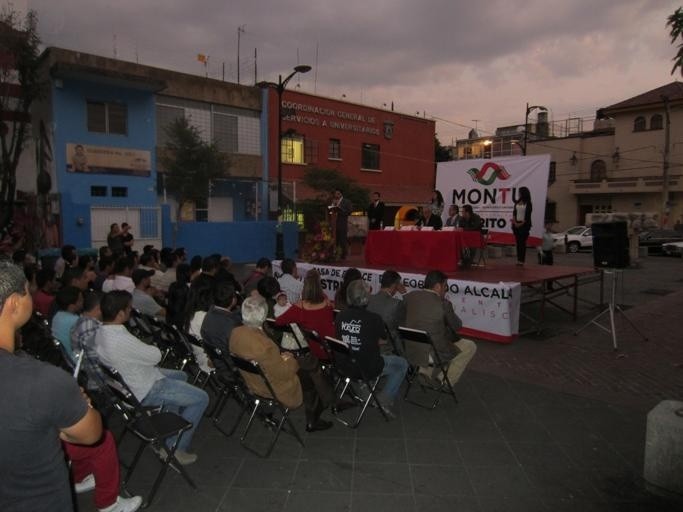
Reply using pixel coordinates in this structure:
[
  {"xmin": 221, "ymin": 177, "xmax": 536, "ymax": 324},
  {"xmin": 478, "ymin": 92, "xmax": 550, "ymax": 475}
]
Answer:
[{"xmin": 575, "ymin": 270, "xmax": 648, "ymax": 349}]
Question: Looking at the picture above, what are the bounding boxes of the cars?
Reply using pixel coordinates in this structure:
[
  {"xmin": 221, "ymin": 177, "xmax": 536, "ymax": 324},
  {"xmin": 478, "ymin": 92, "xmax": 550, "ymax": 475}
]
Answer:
[{"xmin": 548, "ymin": 223, "xmax": 682, "ymax": 257}]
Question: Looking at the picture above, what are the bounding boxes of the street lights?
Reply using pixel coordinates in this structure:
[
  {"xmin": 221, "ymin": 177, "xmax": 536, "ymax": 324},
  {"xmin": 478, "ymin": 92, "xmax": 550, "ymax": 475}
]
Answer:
[
  {"xmin": 510, "ymin": 102, "xmax": 548, "ymax": 155},
  {"xmin": 254, "ymin": 65, "xmax": 312, "ymax": 221}
]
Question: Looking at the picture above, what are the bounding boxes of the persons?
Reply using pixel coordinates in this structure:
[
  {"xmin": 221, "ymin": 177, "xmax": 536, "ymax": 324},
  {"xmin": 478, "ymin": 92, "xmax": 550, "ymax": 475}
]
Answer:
[
  {"xmin": 628, "ymin": 212, "xmax": 683, "ymax": 262},
  {"xmin": 445, "ymin": 205, "xmax": 461, "ymax": 226},
  {"xmin": 366, "ymin": 191, "xmax": 385, "ymax": 230},
  {"xmin": 0, "ymin": 241, "xmax": 479, "ymax": 467},
  {"xmin": 119, "ymin": 222, "xmax": 133, "ymax": 250},
  {"xmin": 425, "ymin": 190, "xmax": 444, "ymax": 229},
  {"xmin": 536, "ymin": 219, "xmax": 559, "ymax": 291},
  {"xmin": 57, "ymin": 426, "xmax": 142, "ymax": 511},
  {"xmin": 66, "ymin": 144, "xmax": 91, "ymax": 173},
  {"xmin": 460, "ymin": 204, "xmax": 481, "ymax": 267},
  {"xmin": 0, "ymin": 259, "xmax": 104, "ymax": 510},
  {"xmin": 106, "ymin": 222, "xmax": 132, "ymax": 250},
  {"xmin": 510, "ymin": 186, "xmax": 532, "ymax": 265},
  {"xmin": 327, "ymin": 188, "xmax": 353, "ymax": 260}
]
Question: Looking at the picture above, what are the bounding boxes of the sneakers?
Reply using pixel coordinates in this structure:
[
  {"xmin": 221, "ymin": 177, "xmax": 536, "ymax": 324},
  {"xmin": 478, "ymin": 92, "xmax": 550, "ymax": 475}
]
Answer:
[
  {"xmin": 74, "ymin": 474, "xmax": 95, "ymax": 493},
  {"xmin": 98, "ymin": 495, "xmax": 143, "ymax": 511},
  {"xmin": 158, "ymin": 446, "xmax": 197, "ymax": 465}
]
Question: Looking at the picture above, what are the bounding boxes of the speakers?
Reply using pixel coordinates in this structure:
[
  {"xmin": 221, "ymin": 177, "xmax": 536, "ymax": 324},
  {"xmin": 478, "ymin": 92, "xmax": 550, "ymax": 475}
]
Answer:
[{"xmin": 592, "ymin": 222, "xmax": 631, "ymax": 269}]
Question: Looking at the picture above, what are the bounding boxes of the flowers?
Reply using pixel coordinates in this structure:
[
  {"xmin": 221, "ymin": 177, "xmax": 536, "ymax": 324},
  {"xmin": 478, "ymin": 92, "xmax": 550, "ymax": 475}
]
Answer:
[{"xmin": 310, "ymin": 221, "xmax": 334, "ymax": 261}]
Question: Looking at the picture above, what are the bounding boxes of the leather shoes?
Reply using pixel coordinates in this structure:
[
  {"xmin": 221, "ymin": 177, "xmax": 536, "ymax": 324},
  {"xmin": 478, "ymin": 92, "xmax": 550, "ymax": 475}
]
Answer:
[
  {"xmin": 307, "ymin": 418, "xmax": 332, "ymax": 432},
  {"xmin": 333, "ymin": 400, "xmax": 355, "ymax": 413}
]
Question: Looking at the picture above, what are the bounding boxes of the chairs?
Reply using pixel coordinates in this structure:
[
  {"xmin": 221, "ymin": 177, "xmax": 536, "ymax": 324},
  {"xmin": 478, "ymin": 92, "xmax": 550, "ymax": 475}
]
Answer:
[
  {"xmin": 22, "ymin": 275, "xmax": 458, "ymax": 512},
  {"xmin": 461, "ymin": 229, "xmax": 488, "ymax": 267}
]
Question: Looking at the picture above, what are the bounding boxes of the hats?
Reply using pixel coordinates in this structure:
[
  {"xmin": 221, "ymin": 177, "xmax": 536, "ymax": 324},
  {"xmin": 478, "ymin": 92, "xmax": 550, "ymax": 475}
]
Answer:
[{"xmin": 133, "ymin": 269, "xmax": 154, "ymax": 284}]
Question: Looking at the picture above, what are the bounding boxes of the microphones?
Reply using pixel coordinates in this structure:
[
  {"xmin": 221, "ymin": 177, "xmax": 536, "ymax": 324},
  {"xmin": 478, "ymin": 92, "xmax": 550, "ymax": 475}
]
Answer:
[{"xmin": 335, "ymin": 196, "xmax": 338, "ymax": 206}]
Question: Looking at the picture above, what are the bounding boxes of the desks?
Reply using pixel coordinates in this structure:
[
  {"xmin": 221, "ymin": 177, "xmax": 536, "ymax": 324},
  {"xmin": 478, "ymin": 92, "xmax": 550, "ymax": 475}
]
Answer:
[{"xmin": 365, "ymin": 228, "xmax": 460, "ymax": 273}]
